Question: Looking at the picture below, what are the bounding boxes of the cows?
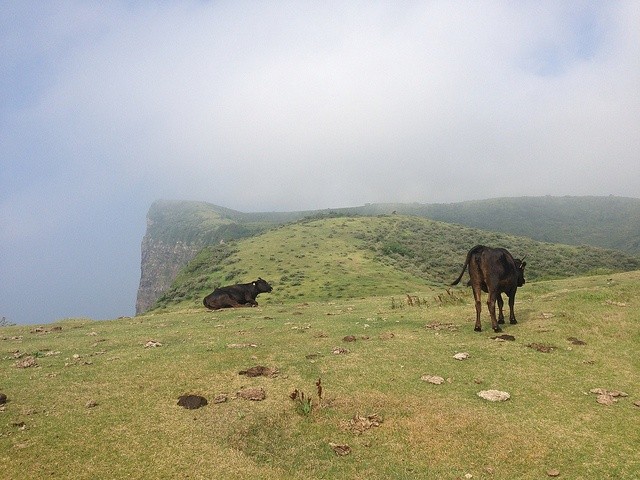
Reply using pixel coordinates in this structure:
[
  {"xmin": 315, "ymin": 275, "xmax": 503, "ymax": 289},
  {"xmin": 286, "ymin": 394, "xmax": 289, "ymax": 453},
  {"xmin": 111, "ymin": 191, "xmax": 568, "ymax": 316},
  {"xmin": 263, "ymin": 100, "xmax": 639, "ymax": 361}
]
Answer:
[
  {"xmin": 450, "ymin": 244, "xmax": 527, "ymax": 334},
  {"xmin": 203, "ymin": 277, "xmax": 274, "ymax": 310}
]
